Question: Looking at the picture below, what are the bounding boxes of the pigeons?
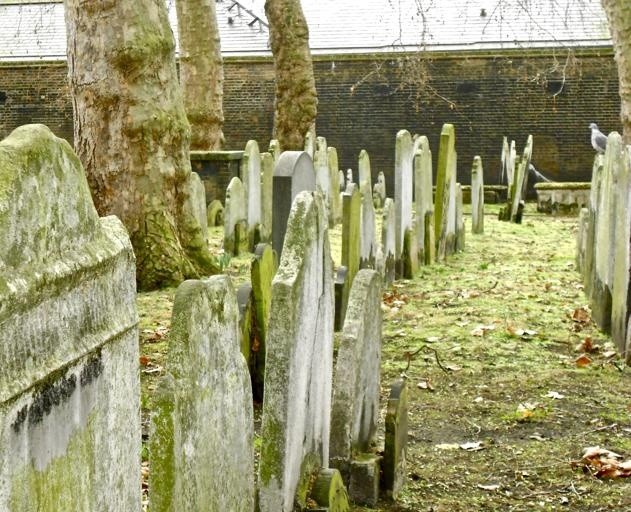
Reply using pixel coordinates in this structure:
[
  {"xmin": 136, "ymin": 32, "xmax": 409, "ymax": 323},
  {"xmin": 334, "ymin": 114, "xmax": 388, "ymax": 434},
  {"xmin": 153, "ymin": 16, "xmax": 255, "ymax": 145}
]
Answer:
[{"xmin": 588, "ymin": 122, "xmax": 607, "ymax": 153}]
